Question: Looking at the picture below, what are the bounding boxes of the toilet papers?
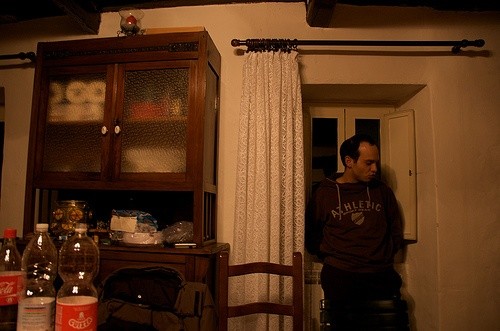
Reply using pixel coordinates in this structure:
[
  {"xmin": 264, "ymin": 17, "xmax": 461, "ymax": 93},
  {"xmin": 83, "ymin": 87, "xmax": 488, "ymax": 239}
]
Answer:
[
  {"xmin": 48, "ymin": 104, "xmax": 104, "ymax": 121},
  {"xmin": 88, "ymin": 81, "xmax": 106, "ymax": 104},
  {"xmin": 50, "ymin": 82, "xmax": 63, "ymax": 103},
  {"xmin": 65, "ymin": 81, "xmax": 88, "ymax": 103}
]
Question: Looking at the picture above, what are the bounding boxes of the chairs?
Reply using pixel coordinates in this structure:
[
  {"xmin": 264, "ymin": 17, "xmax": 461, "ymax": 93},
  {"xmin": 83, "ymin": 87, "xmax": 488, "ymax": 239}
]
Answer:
[{"xmin": 215, "ymin": 252, "xmax": 305, "ymax": 331}]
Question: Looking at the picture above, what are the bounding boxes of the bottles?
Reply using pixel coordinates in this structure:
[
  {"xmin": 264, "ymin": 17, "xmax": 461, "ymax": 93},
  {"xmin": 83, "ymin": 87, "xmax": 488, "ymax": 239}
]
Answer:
[
  {"xmin": 17, "ymin": 223, "xmax": 58, "ymax": 331},
  {"xmin": 0, "ymin": 229, "xmax": 22, "ymax": 331},
  {"xmin": 55, "ymin": 223, "xmax": 100, "ymax": 331}
]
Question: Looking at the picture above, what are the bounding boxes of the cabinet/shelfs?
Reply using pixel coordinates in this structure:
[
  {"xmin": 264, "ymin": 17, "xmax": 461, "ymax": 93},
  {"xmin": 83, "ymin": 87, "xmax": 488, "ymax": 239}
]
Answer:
[{"xmin": 24, "ymin": 29, "xmax": 230, "ymax": 331}]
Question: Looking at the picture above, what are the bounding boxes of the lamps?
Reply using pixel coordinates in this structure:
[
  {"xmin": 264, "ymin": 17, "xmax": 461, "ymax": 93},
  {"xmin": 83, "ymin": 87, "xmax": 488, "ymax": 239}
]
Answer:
[{"xmin": 116, "ymin": 7, "xmax": 146, "ymax": 35}]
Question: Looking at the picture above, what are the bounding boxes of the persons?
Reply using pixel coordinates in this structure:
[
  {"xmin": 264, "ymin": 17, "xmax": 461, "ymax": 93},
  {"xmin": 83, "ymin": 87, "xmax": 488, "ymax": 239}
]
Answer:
[{"xmin": 304, "ymin": 134, "xmax": 410, "ymax": 331}]
